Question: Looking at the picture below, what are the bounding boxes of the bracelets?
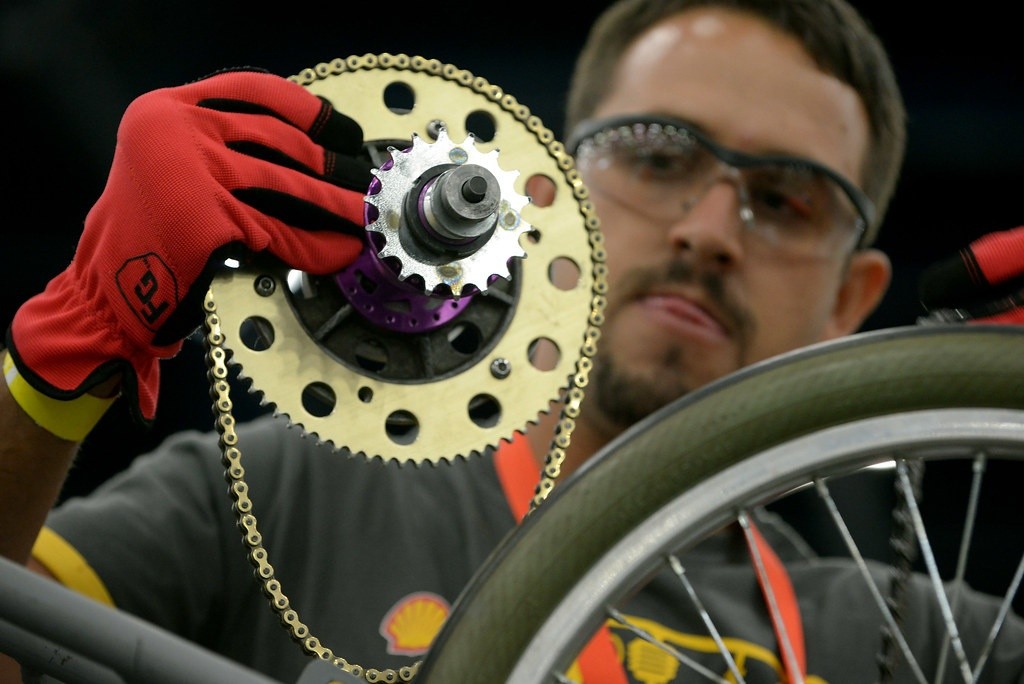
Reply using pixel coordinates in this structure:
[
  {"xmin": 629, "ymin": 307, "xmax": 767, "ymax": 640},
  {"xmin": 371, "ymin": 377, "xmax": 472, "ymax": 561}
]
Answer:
[{"xmin": 1, "ymin": 349, "xmax": 118, "ymax": 445}]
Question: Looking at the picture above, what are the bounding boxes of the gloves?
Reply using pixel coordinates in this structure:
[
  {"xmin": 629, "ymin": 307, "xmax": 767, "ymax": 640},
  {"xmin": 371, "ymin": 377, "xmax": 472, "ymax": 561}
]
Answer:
[
  {"xmin": 945, "ymin": 226, "xmax": 1024, "ymax": 324},
  {"xmin": 8, "ymin": 73, "xmax": 371, "ymax": 428}
]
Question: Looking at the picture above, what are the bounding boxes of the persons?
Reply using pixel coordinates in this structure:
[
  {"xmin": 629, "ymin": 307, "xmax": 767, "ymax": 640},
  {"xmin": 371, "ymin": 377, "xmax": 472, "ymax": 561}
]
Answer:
[{"xmin": 0, "ymin": 1, "xmax": 1024, "ymax": 684}]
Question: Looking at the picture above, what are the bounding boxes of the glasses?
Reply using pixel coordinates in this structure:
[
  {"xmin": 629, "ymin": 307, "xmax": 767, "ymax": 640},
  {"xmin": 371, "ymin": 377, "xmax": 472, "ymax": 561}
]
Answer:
[{"xmin": 565, "ymin": 112, "xmax": 877, "ymax": 260}]
